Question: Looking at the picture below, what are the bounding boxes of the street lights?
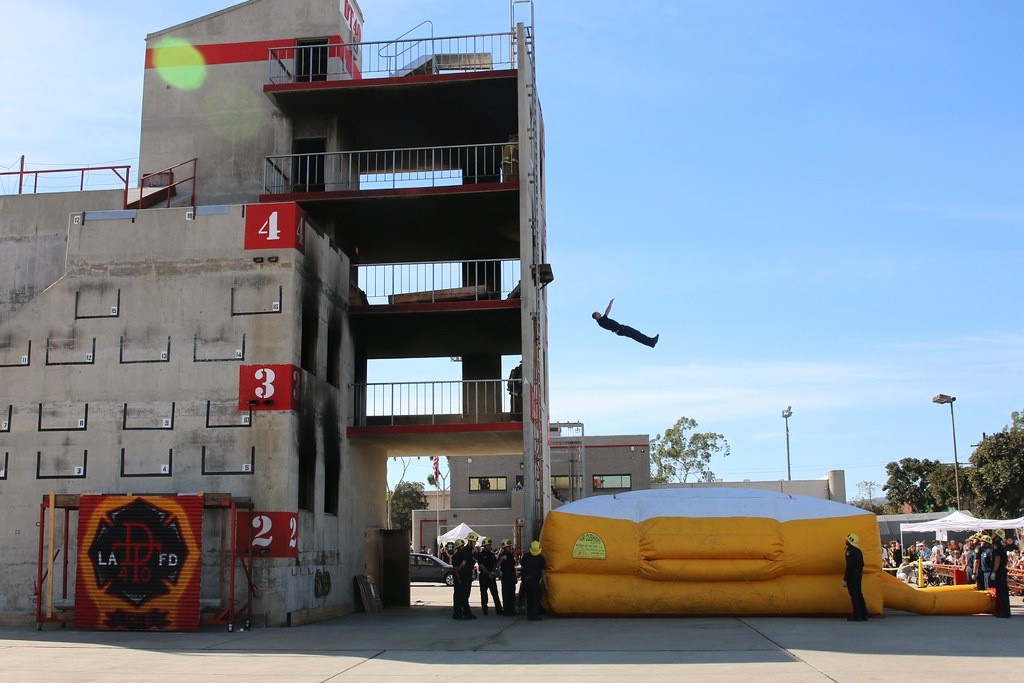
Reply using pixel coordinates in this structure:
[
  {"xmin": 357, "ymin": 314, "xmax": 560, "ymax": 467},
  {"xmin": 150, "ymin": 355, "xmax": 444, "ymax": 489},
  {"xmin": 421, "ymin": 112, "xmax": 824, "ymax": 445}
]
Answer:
[
  {"xmin": 782, "ymin": 406, "xmax": 792, "ymax": 480},
  {"xmin": 932, "ymin": 394, "xmax": 960, "ymax": 511}
]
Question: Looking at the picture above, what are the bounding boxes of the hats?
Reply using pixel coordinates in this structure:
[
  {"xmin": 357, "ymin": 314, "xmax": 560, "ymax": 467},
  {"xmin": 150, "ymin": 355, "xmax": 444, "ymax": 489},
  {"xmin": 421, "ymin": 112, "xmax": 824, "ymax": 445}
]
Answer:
[
  {"xmin": 980, "ymin": 535, "xmax": 992, "ymax": 545},
  {"xmin": 846, "ymin": 533, "xmax": 858, "ymax": 547},
  {"xmin": 959, "ymin": 539, "xmax": 963, "ymax": 543},
  {"xmin": 993, "ymin": 529, "xmax": 1006, "ymax": 542},
  {"xmin": 964, "ymin": 533, "xmax": 983, "ymax": 543},
  {"xmin": 888, "ymin": 540, "xmax": 896, "ymax": 546},
  {"xmin": 931, "ymin": 539, "xmax": 936, "ymax": 544},
  {"xmin": 950, "ymin": 540, "xmax": 955, "ymax": 543}
]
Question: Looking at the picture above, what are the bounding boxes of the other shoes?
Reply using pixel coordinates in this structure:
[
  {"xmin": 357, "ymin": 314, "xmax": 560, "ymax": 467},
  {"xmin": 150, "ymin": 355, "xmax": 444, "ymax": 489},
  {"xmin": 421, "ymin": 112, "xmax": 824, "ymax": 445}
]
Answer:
[
  {"xmin": 848, "ymin": 614, "xmax": 869, "ymax": 621},
  {"xmin": 526, "ymin": 613, "xmax": 543, "ymax": 621},
  {"xmin": 482, "ymin": 611, "xmax": 488, "ymax": 615},
  {"xmin": 943, "ymin": 582, "xmax": 954, "ymax": 586},
  {"xmin": 496, "ymin": 609, "xmax": 503, "ymax": 615},
  {"xmin": 451, "ymin": 612, "xmax": 477, "ymax": 620}
]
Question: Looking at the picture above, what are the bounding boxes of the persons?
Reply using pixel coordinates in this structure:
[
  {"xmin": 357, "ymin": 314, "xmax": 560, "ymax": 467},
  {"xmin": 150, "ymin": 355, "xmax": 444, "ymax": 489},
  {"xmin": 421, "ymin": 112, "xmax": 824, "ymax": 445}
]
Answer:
[
  {"xmin": 989, "ymin": 529, "xmax": 1012, "ymax": 618},
  {"xmin": 495, "ymin": 538, "xmax": 517, "ymax": 616},
  {"xmin": 842, "ymin": 533, "xmax": 869, "ymax": 620},
  {"xmin": 501, "ymin": 134, "xmax": 520, "ymax": 182},
  {"xmin": 410, "ymin": 541, "xmax": 524, "ymax": 580},
  {"xmin": 896, "ymin": 559, "xmax": 914, "ymax": 583},
  {"xmin": 478, "ymin": 538, "xmax": 503, "ymax": 615},
  {"xmin": 519, "ymin": 541, "xmax": 553, "ymax": 617},
  {"xmin": 591, "ymin": 298, "xmax": 659, "ymax": 348},
  {"xmin": 881, "ymin": 534, "xmax": 1024, "ymax": 596},
  {"xmin": 451, "ymin": 532, "xmax": 478, "ymax": 619}
]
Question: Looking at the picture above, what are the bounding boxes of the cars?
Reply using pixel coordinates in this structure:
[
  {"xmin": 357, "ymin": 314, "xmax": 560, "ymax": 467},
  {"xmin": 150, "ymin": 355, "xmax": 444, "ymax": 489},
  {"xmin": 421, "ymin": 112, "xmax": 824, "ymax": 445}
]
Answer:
[{"xmin": 409, "ymin": 554, "xmax": 478, "ymax": 586}]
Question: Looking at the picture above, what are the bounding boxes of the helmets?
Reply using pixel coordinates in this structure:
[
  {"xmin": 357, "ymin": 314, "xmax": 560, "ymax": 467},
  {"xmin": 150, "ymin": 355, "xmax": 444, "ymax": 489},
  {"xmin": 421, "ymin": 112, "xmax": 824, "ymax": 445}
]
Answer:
[
  {"xmin": 529, "ymin": 540, "xmax": 542, "ymax": 557},
  {"xmin": 481, "ymin": 538, "xmax": 492, "ymax": 548},
  {"xmin": 464, "ymin": 532, "xmax": 478, "ymax": 543},
  {"xmin": 454, "ymin": 539, "xmax": 465, "ymax": 550},
  {"xmin": 501, "ymin": 538, "xmax": 512, "ymax": 549}
]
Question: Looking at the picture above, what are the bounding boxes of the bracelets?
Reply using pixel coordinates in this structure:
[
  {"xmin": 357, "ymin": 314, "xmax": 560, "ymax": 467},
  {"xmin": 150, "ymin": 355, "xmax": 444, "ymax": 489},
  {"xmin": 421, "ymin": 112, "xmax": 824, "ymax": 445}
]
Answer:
[
  {"xmin": 972, "ymin": 572, "xmax": 976, "ymax": 575},
  {"xmin": 993, "ymin": 571, "xmax": 996, "ymax": 572}
]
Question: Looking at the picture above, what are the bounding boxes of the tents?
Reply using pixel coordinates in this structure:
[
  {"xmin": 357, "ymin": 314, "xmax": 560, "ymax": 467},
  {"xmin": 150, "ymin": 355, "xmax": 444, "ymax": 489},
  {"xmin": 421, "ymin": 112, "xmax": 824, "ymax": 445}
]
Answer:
[
  {"xmin": 436, "ymin": 520, "xmax": 486, "ymax": 547},
  {"xmin": 899, "ymin": 510, "xmax": 1024, "ymax": 564}
]
